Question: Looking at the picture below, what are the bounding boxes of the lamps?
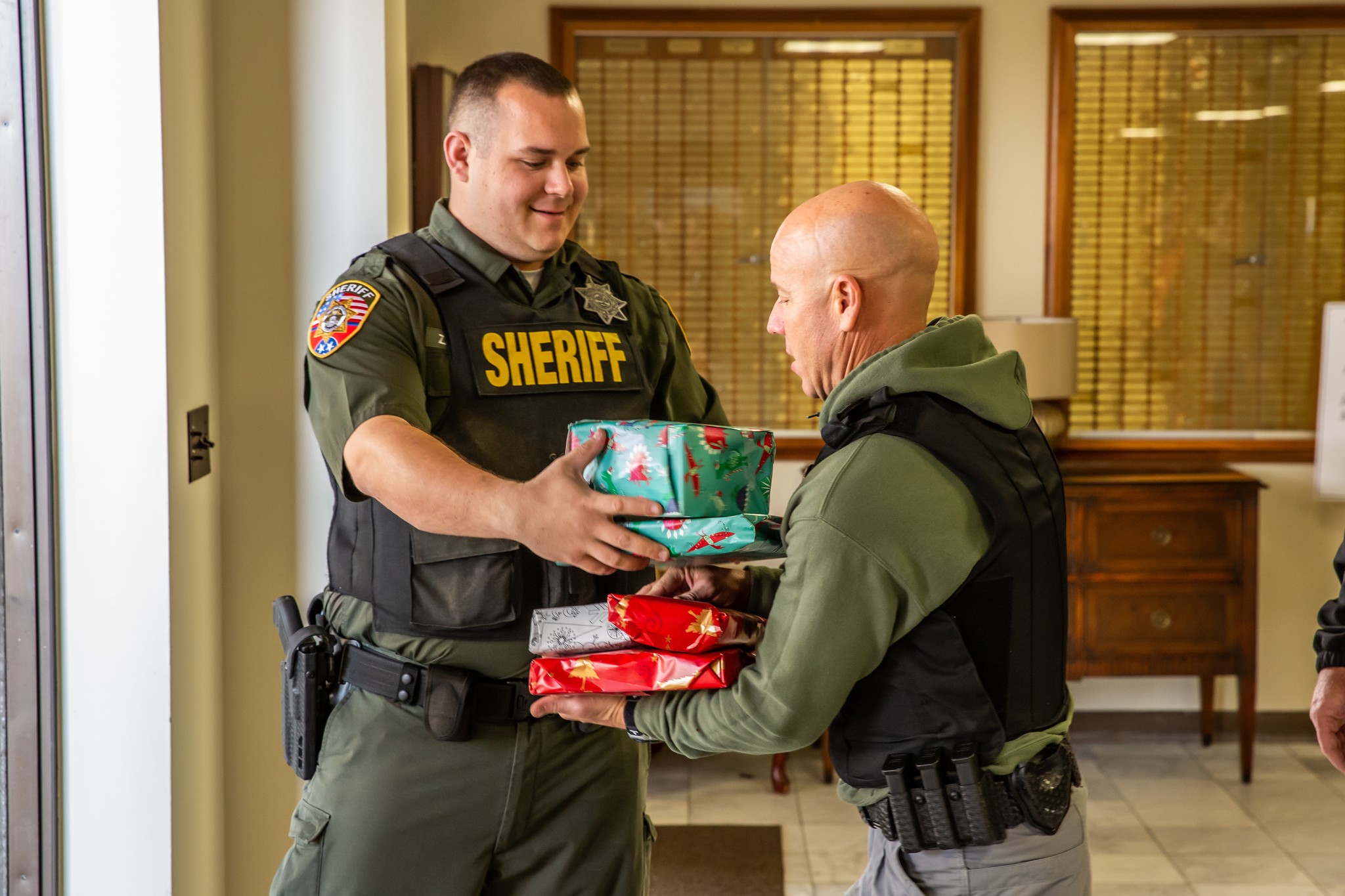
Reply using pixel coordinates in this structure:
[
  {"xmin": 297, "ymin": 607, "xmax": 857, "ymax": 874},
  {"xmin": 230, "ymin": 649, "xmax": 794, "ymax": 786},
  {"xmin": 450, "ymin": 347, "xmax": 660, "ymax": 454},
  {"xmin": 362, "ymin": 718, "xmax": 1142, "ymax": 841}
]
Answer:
[{"xmin": 980, "ymin": 318, "xmax": 1078, "ymax": 443}]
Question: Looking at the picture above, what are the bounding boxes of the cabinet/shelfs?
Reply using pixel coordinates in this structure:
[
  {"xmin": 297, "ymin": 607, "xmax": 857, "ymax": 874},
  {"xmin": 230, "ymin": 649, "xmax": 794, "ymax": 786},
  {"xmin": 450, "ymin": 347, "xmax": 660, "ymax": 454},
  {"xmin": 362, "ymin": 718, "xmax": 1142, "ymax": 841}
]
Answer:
[{"xmin": 1059, "ymin": 448, "xmax": 1267, "ymax": 787}]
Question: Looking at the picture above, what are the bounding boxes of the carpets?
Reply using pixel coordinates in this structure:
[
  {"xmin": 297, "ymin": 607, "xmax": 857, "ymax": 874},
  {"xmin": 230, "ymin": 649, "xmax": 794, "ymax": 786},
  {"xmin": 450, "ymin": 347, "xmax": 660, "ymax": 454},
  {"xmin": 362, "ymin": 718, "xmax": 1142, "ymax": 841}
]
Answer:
[{"xmin": 645, "ymin": 822, "xmax": 783, "ymax": 896}]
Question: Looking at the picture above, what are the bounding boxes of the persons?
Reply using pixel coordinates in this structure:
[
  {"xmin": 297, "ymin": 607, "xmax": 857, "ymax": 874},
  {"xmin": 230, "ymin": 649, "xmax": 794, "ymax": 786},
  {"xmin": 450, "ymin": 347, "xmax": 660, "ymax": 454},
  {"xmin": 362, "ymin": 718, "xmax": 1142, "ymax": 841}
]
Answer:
[
  {"xmin": 265, "ymin": 50, "xmax": 732, "ymax": 895},
  {"xmin": 528, "ymin": 179, "xmax": 1096, "ymax": 896}
]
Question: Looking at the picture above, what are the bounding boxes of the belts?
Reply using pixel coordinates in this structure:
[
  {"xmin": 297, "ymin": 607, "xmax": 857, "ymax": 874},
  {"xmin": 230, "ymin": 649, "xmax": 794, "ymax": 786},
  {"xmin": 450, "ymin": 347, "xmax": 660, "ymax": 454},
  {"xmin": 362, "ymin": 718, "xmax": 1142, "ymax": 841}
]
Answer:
[{"xmin": 331, "ymin": 645, "xmax": 535, "ymax": 730}]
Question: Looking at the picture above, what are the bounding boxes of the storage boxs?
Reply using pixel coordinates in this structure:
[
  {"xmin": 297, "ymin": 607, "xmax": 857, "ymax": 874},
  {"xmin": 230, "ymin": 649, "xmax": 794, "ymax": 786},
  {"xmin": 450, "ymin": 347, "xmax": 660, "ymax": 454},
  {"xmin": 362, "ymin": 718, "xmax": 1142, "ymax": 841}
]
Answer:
[
  {"xmin": 527, "ymin": 601, "xmax": 639, "ymax": 655},
  {"xmin": 554, "ymin": 516, "xmax": 793, "ymax": 564},
  {"xmin": 608, "ymin": 596, "xmax": 773, "ymax": 652},
  {"xmin": 528, "ymin": 642, "xmax": 752, "ymax": 696},
  {"xmin": 563, "ymin": 415, "xmax": 779, "ymax": 518}
]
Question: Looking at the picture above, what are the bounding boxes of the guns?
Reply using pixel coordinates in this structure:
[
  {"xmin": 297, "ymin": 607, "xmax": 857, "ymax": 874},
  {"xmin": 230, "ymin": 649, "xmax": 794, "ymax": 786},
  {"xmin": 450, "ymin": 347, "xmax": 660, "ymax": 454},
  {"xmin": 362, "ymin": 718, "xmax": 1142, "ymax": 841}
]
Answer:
[{"xmin": 270, "ymin": 593, "xmax": 326, "ymax": 786}]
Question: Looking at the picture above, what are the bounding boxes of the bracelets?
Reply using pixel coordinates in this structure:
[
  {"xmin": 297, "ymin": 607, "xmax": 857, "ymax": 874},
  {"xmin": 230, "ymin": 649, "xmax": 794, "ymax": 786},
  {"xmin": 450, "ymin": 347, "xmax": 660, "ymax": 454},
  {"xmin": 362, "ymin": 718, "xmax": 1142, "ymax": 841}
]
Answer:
[{"xmin": 622, "ymin": 695, "xmax": 660, "ymax": 743}]
